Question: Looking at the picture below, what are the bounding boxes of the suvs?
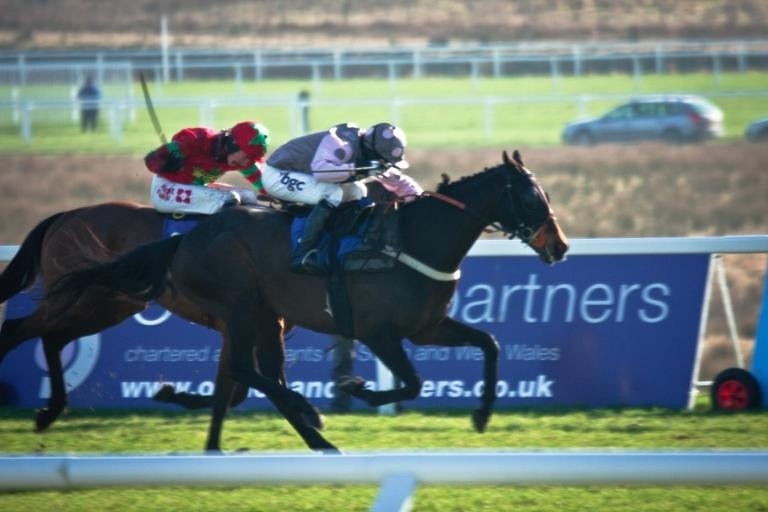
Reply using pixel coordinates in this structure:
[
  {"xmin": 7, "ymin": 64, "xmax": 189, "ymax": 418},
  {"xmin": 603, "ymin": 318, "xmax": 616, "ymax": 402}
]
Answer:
[{"xmin": 562, "ymin": 95, "xmax": 724, "ymax": 147}]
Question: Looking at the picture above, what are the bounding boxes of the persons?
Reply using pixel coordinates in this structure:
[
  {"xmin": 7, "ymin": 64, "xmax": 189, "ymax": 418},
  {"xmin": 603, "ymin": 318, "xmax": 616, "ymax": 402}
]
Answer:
[
  {"xmin": 144, "ymin": 120, "xmax": 269, "ymax": 214},
  {"xmin": 77, "ymin": 78, "xmax": 101, "ymax": 133},
  {"xmin": 259, "ymin": 124, "xmax": 423, "ymax": 272}
]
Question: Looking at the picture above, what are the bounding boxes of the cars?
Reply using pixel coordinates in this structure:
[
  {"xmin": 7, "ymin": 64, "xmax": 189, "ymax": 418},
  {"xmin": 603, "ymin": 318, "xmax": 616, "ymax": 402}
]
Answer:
[{"xmin": 744, "ymin": 120, "xmax": 768, "ymax": 141}]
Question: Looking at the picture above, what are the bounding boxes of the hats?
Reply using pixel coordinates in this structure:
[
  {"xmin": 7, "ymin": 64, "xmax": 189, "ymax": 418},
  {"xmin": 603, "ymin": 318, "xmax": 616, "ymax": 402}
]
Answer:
[
  {"xmin": 231, "ymin": 121, "xmax": 270, "ymax": 164},
  {"xmin": 364, "ymin": 122, "xmax": 410, "ymax": 170}
]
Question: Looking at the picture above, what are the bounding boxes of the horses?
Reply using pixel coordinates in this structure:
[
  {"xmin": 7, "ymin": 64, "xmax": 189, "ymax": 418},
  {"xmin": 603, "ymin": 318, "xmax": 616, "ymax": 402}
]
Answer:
[
  {"xmin": 0, "ymin": 180, "xmax": 405, "ymax": 448},
  {"xmin": 40, "ymin": 148, "xmax": 571, "ymax": 455}
]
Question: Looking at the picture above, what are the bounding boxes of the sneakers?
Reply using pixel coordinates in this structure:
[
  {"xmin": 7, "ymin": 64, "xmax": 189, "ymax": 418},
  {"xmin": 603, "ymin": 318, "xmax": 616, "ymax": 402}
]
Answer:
[{"xmin": 287, "ymin": 255, "xmax": 329, "ymax": 276}]
